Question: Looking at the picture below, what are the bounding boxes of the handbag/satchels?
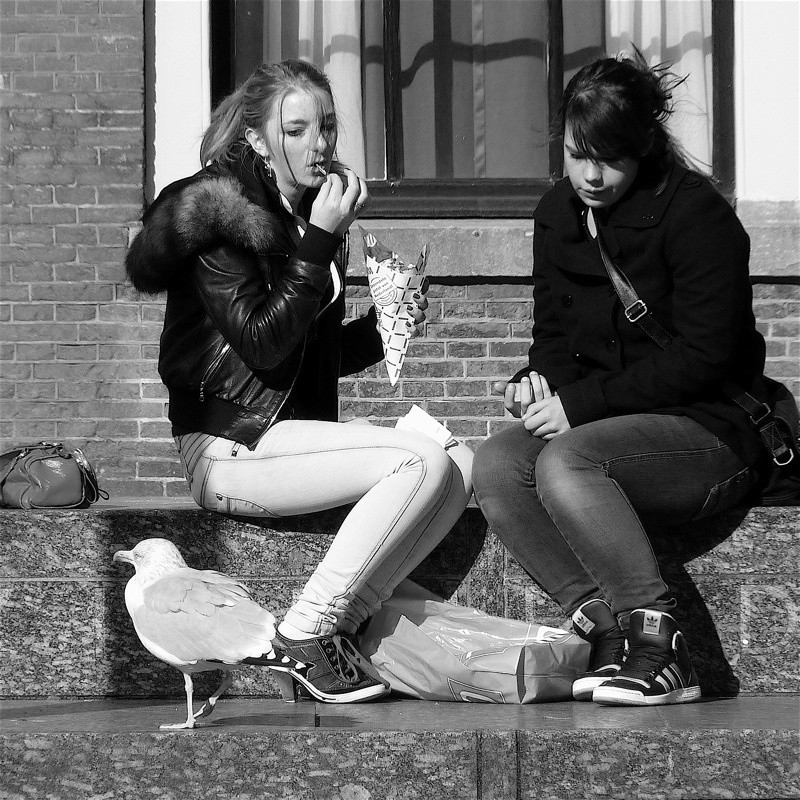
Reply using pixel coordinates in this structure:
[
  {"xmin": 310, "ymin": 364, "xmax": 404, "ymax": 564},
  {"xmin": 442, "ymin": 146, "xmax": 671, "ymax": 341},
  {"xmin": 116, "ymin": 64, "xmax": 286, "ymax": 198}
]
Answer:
[
  {"xmin": 753, "ymin": 374, "xmax": 800, "ymax": 506},
  {"xmin": 358, "ymin": 576, "xmax": 590, "ymax": 704},
  {"xmin": 0, "ymin": 440, "xmax": 109, "ymax": 511}
]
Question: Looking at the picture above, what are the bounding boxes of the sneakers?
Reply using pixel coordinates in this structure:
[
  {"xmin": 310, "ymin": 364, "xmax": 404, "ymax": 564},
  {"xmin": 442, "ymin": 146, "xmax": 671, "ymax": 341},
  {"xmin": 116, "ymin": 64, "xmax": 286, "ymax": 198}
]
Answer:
[
  {"xmin": 271, "ymin": 627, "xmax": 390, "ymax": 704},
  {"xmin": 592, "ymin": 608, "xmax": 701, "ymax": 706},
  {"xmin": 571, "ymin": 598, "xmax": 628, "ymax": 699}
]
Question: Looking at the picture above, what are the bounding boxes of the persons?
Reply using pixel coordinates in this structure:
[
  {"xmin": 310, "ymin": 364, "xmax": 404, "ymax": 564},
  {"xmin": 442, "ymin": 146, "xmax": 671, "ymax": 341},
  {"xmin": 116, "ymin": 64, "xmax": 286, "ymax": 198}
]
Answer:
[
  {"xmin": 472, "ymin": 58, "xmax": 768, "ymax": 707},
  {"xmin": 125, "ymin": 61, "xmax": 474, "ymax": 705}
]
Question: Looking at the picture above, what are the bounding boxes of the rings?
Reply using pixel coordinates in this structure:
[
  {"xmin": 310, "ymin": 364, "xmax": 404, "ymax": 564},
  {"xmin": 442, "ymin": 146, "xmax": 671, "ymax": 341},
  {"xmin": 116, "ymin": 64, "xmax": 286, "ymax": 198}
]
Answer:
[{"xmin": 354, "ymin": 202, "xmax": 365, "ymax": 210}]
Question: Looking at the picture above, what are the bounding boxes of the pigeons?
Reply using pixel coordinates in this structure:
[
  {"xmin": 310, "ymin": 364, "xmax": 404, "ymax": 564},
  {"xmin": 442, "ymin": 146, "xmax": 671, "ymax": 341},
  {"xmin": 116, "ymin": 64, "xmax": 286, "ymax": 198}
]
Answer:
[{"xmin": 115, "ymin": 537, "xmax": 276, "ymax": 730}]
